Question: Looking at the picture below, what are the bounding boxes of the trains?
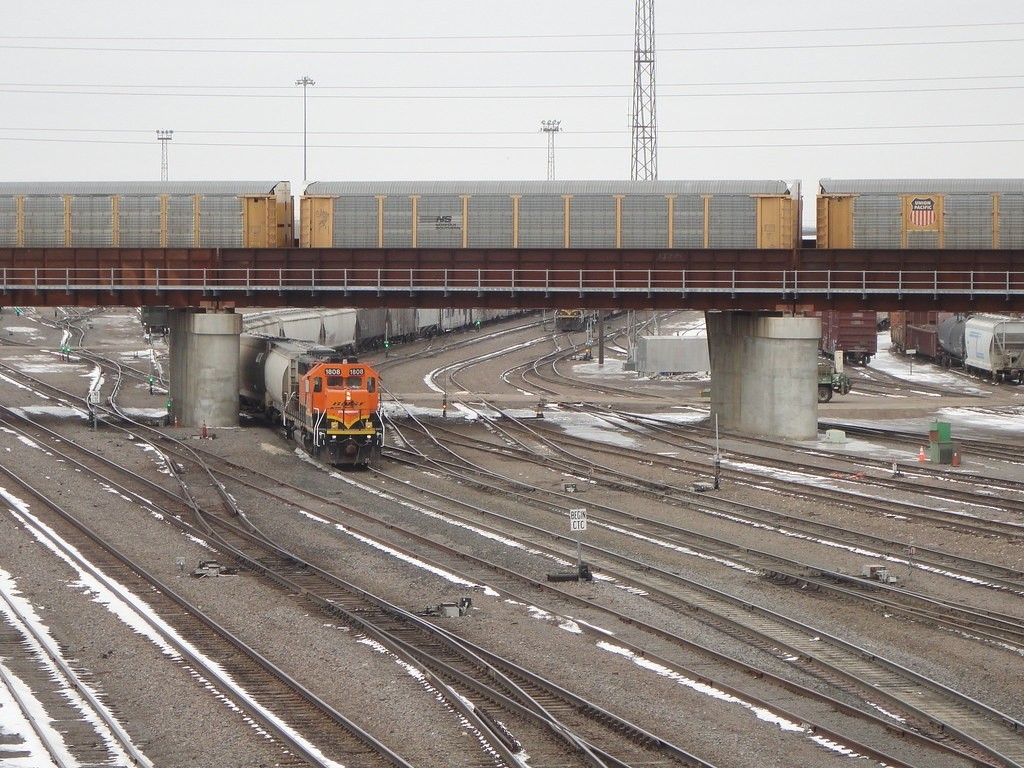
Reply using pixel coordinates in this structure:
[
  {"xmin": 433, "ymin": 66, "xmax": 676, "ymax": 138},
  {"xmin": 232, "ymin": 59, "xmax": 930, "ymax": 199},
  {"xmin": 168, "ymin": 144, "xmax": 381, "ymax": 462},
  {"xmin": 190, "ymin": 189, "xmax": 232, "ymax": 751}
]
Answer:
[
  {"xmin": 556, "ymin": 306, "xmax": 637, "ymax": 332},
  {"xmin": 888, "ymin": 311, "xmax": 1024, "ymax": 383},
  {"xmin": 237, "ymin": 331, "xmax": 386, "ymax": 475},
  {"xmin": 819, "ymin": 308, "xmax": 878, "ymax": 362},
  {"xmin": 140, "ymin": 306, "xmax": 173, "ymax": 335}
]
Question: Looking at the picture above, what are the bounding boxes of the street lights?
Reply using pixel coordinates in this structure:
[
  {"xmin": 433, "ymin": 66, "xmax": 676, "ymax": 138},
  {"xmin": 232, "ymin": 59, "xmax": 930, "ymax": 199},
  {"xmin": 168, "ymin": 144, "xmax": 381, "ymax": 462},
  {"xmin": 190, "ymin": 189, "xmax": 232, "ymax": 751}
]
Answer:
[{"xmin": 295, "ymin": 74, "xmax": 317, "ymax": 182}]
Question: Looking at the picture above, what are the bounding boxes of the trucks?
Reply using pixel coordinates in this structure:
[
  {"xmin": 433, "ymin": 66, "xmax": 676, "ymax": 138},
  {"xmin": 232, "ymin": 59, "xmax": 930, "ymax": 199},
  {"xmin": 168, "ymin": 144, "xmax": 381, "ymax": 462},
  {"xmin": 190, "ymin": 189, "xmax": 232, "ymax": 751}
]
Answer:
[{"xmin": 818, "ymin": 363, "xmax": 852, "ymax": 404}]
